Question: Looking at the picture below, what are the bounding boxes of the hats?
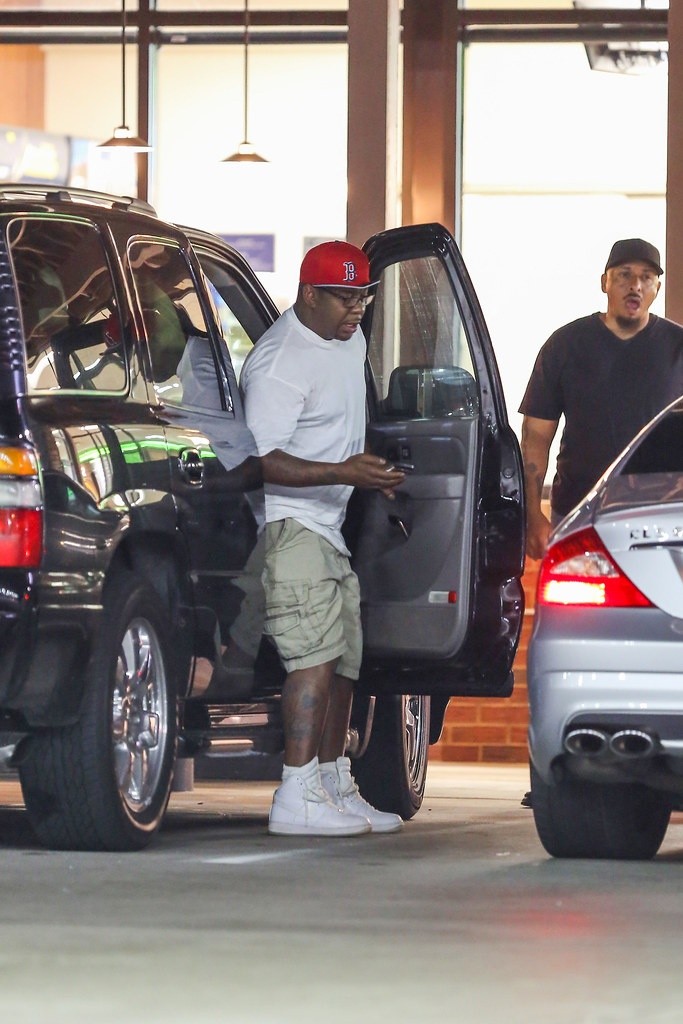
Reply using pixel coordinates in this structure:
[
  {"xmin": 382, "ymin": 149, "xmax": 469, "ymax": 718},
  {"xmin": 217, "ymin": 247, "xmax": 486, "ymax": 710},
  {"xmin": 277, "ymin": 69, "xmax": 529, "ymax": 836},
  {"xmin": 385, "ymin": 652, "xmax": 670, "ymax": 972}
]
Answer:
[
  {"xmin": 604, "ymin": 238, "xmax": 664, "ymax": 277},
  {"xmin": 300, "ymin": 241, "xmax": 380, "ymax": 288}
]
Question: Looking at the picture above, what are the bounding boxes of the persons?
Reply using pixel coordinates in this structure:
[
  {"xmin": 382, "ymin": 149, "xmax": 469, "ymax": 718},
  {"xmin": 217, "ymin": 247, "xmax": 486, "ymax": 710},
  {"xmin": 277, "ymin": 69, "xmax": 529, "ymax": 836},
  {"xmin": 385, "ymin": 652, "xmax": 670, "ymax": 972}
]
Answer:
[
  {"xmin": 100, "ymin": 307, "xmax": 265, "ymax": 702},
  {"xmin": 239, "ymin": 240, "xmax": 406, "ymax": 836},
  {"xmin": 518, "ymin": 239, "xmax": 683, "ymax": 561}
]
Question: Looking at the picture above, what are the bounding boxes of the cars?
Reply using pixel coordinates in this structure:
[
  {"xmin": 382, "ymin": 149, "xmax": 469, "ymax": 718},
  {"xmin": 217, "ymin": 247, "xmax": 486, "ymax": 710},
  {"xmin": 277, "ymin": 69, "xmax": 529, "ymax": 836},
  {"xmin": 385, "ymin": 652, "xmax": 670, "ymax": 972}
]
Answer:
[{"xmin": 524, "ymin": 393, "xmax": 683, "ymax": 859}]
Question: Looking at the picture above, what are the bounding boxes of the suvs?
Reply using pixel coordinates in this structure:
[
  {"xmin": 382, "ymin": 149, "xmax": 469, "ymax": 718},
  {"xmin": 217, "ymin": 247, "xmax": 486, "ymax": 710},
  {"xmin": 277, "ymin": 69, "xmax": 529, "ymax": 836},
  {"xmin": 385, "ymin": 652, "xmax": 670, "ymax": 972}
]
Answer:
[{"xmin": 0, "ymin": 179, "xmax": 530, "ymax": 850}]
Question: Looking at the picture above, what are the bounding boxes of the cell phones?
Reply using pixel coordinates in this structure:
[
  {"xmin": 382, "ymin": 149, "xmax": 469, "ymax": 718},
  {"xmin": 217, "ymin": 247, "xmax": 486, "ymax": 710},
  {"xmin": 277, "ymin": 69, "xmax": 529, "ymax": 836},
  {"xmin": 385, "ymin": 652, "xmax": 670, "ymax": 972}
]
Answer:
[{"xmin": 384, "ymin": 463, "xmax": 415, "ymax": 475}]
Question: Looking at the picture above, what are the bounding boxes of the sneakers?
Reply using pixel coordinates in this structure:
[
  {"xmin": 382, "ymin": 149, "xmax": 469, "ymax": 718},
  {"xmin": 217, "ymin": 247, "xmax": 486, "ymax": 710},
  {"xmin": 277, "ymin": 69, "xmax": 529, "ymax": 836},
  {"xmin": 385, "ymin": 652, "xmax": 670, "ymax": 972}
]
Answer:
[
  {"xmin": 320, "ymin": 756, "xmax": 405, "ymax": 832},
  {"xmin": 268, "ymin": 756, "xmax": 371, "ymax": 835}
]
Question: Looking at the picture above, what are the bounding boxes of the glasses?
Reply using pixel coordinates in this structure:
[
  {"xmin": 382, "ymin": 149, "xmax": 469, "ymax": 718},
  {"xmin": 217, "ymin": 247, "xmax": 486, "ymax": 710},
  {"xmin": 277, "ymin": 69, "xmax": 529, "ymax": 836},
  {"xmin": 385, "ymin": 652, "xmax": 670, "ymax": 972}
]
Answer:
[{"xmin": 321, "ymin": 287, "xmax": 377, "ymax": 308}]
